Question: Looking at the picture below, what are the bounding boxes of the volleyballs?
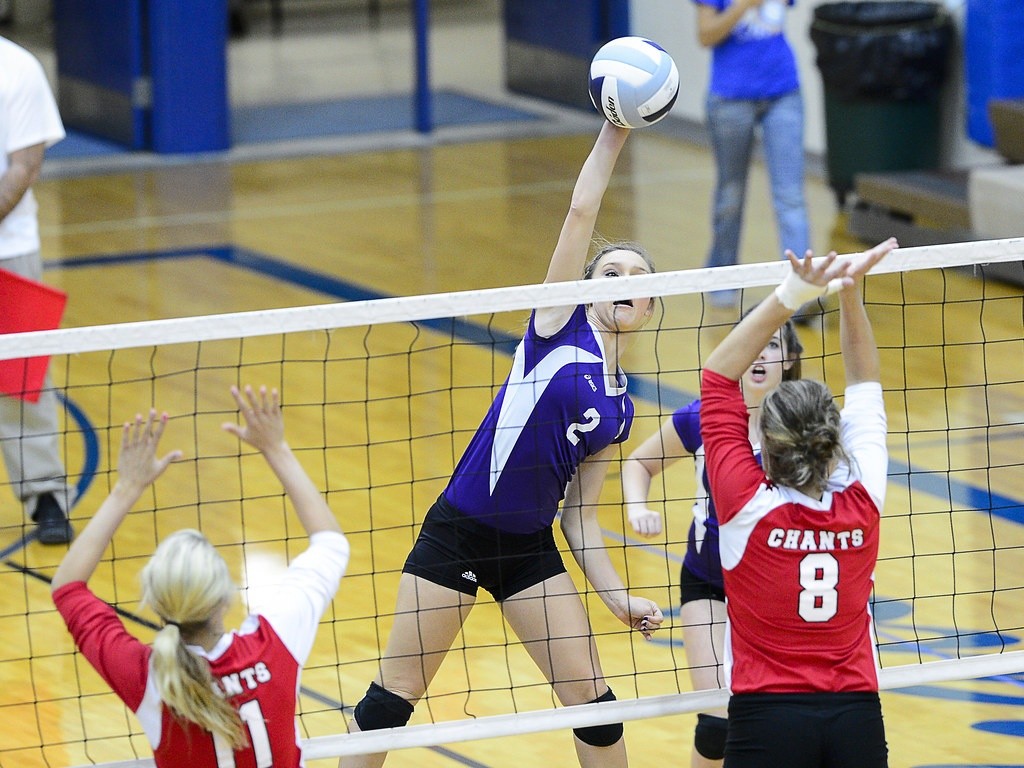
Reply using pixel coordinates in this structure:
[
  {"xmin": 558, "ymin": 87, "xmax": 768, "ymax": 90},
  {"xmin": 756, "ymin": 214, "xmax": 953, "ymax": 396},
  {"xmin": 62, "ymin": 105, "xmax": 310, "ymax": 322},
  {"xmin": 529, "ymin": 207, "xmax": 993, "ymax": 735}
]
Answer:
[{"xmin": 585, "ymin": 36, "xmax": 683, "ymax": 131}]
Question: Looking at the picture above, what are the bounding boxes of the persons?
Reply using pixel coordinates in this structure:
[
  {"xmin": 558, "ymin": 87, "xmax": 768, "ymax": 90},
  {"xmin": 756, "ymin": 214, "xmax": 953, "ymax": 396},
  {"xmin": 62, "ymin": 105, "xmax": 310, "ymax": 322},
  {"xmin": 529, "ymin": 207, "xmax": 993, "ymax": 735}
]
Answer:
[
  {"xmin": 622, "ymin": 230, "xmax": 901, "ymax": 768},
  {"xmin": 47, "ymin": 381, "xmax": 350, "ymax": 768},
  {"xmin": 336, "ymin": 113, "xmax": 665, "ymax": 768},
  {"xmin": 0, "ymin": 40, "xmax": 98, "ymax": 549},
  {"xmin": 696, "ymin": 0, "xmax": 828, "ymax": 329}
]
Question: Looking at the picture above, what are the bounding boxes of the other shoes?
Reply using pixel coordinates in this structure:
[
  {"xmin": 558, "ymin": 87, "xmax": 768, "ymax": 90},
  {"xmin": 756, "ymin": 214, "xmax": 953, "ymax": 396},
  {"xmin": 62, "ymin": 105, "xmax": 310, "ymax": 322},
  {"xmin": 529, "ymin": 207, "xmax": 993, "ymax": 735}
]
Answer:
[
  {"xmin": 791, "ymin": 296, "xmax": 826, "ymax": 327},
  {"xmin": 707, "ymin": 290, "xmax": 740, "ymax": 309}
]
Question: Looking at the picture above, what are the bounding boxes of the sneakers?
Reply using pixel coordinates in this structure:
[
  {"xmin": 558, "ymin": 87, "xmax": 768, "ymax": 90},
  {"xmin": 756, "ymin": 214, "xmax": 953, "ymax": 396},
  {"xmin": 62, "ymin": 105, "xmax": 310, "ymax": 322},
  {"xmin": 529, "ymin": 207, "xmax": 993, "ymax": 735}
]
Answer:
[{"xmin": 31, "ymin": 492, "xmax": 73, "ymax": 544}]
